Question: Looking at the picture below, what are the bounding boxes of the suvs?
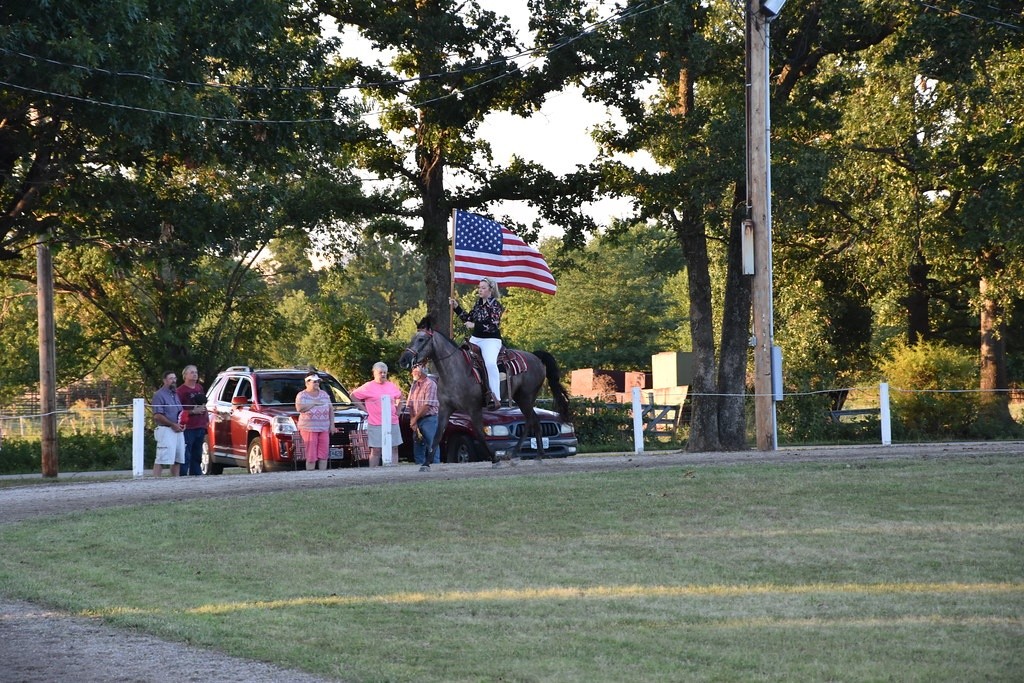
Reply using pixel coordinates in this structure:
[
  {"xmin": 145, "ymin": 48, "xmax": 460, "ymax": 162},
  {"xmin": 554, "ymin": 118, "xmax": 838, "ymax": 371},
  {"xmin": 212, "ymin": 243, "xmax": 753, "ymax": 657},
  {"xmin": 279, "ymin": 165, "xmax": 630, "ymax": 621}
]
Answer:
[{"xmin": 200, "ymin": 363, "xmax": 369, "ymax": 475}]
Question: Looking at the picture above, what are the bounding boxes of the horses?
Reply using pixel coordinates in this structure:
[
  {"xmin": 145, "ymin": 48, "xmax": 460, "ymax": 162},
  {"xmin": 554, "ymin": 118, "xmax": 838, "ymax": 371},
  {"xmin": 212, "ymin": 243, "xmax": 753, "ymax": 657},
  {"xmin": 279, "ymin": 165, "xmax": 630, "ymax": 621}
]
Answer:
[{"xmin": 397, "ymin": 319, "xmax": 571, "ymax": 472}]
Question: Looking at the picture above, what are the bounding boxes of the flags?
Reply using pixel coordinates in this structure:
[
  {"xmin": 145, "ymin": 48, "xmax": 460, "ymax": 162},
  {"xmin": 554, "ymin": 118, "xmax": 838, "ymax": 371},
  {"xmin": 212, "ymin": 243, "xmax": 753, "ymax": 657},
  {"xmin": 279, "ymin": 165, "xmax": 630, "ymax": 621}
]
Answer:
[{"xmin": 453, "ymin": 209, "xmax": 557, "ymax": 296}]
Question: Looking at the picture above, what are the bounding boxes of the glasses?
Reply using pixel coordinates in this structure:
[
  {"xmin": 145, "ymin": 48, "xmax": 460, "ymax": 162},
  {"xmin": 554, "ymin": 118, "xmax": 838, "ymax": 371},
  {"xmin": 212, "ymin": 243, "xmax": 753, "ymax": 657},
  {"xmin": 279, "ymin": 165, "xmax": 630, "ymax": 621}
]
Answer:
[
  {"xmin": 313, "ymin": 380, "xmax": 320, "ymax": 384},
  {"xmin": 481, "ymin": 279, "xmax": 492, "ymax": 288},
  {"xmin": 165, "ymin": 377, "xmax": 177, "ymax": 380}
]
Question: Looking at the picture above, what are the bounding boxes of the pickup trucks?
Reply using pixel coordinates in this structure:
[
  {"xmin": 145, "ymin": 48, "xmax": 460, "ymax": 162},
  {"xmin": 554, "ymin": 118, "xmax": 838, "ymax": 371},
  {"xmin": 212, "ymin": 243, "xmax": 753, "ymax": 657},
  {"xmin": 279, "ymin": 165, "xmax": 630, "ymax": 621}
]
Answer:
[{"xmin": 395, "ymin": 374, "xmax": 578, "ymax": 462}]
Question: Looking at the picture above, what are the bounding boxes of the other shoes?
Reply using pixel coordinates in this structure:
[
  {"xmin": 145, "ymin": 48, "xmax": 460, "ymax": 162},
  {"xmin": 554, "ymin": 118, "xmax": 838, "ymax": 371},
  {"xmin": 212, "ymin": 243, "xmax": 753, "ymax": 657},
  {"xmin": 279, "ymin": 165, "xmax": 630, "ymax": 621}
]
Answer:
[{"xmin": 488, "ymin": 398, "xmax": 502, "ymax": 408}]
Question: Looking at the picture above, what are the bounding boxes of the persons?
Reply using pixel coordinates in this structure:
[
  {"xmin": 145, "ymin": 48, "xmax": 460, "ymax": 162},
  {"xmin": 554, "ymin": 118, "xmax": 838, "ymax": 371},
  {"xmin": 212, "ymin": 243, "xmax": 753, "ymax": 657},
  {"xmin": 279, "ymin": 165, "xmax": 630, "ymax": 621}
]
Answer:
[
  {"xmin": 295, "ymin": 371, "xmax": 335, "ymax": 471},
  {"xmin": 259, "ymin": 385, "xmax": 282, "ymax": 405},
  {"xmin": 448, "ymin": 277, "xmax": 504, "ymax": 411},
  {"xmin": 176, "ymin": 364, "xmax": 210, "ymax": 476},
  {"xmin": 349, "ymin": 362, "xmax": 404, "ymax": 466},
  {"xmin": 405, "ymin": 365, "xmax": 440, "ymax": 464},
  {"xmin": 152, "ymin": 370, "xmax": 187, "ymax": 477}
]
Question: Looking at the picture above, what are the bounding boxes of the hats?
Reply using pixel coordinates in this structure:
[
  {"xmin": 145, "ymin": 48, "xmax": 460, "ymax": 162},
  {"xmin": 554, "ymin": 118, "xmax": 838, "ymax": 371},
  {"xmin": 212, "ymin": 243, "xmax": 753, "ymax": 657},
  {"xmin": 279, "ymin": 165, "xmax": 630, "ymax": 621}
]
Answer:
[
  {"xmin": 177, "ymin": 410, "xmax": 190, "ymax": 432},
  {"xmin": 414, "ymin": 427, "xmax": 426, "ymax": 444},
  {"xmin": 305, "ymin": 374, "xmax": 322, "ymax": 382}
]
